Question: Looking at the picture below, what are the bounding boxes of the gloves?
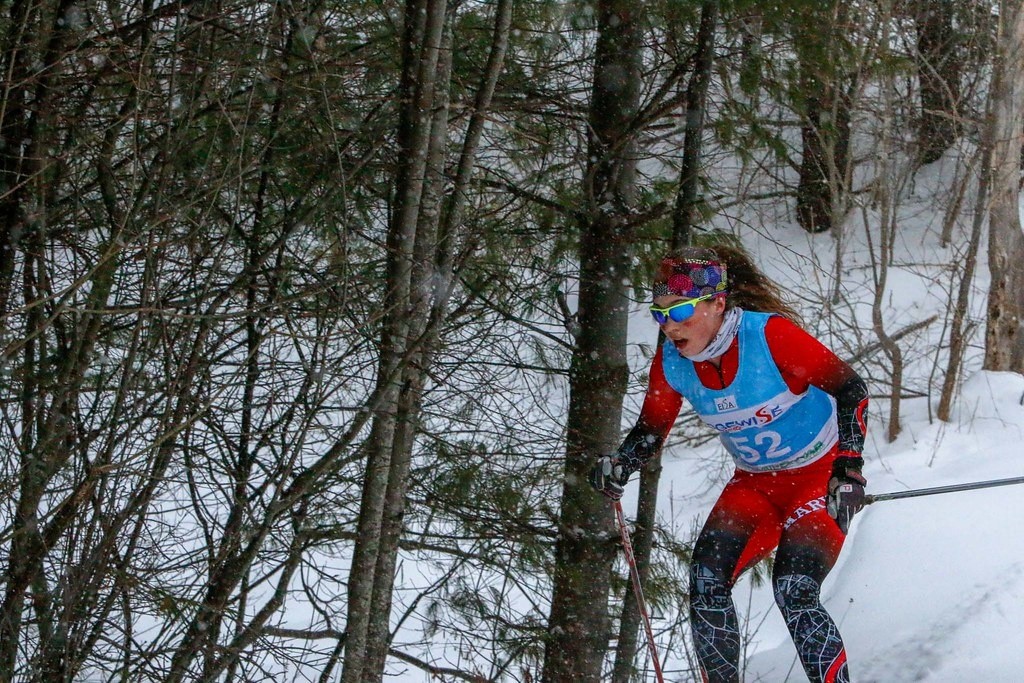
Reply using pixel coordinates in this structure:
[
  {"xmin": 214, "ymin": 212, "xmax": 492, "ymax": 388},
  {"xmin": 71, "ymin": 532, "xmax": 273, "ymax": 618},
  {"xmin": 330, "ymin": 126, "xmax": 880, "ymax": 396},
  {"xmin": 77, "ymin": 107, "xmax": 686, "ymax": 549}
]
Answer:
[
  {"xmin": 826, "ymin": 456, "xmax": 867, "ymax": 536},
  {"xmin": 590, "ymin": 449, "xmax": 642, "ymax": 500}
]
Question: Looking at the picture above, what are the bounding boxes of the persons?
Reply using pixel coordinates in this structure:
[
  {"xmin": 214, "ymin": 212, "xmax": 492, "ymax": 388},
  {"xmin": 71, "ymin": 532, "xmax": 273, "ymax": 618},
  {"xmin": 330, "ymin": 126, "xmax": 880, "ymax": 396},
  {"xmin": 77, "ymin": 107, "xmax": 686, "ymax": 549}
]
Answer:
[{"xmin": 589, "ymin": 247, "xmax": 868, "ymax": 683}]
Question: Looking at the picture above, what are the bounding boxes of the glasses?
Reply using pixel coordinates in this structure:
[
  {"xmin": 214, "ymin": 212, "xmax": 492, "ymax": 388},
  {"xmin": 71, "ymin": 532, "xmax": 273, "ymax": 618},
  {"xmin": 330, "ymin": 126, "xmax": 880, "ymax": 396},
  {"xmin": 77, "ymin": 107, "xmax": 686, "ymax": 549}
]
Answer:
[{"xmin": 649, "ymin": 290, "xmax": 727, "ymax": 324}]
